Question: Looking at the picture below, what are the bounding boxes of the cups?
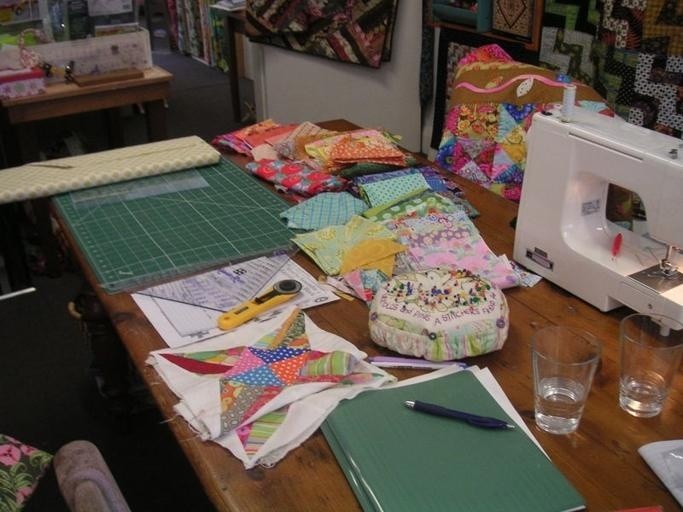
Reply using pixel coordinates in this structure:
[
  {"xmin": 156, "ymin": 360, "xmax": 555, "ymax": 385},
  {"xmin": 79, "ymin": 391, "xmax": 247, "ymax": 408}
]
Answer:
[
  {"xmin": 530, "ymin": 322, "xmax": 602, "ymax": 436},
  {"xmin": 616, "ymin": 310, "xmax": 683, "ymax": 419}
]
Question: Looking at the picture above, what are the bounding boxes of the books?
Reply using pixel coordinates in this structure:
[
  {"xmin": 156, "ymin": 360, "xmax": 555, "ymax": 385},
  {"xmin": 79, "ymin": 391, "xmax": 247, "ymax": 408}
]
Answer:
[{"xmin": 321, "ymin": 363, "xmax": 586, "ymax": 512}]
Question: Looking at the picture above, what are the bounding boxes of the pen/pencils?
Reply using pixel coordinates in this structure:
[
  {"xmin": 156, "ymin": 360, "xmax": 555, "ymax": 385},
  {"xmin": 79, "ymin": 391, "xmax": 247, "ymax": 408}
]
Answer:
[{"xmin": 406, "ymin": 400, "xmax": 517, "ymax": 435}]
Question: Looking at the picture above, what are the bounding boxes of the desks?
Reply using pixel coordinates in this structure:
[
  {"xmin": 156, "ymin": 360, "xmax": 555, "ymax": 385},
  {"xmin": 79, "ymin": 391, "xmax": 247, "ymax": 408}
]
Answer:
[
  {"xmin": 36, "ymin": 120, "xmax": 679, "ymax": 512},
  {"xmin": 1, "ymin": 39, "xmax": 175, "ymax": 166}
]
